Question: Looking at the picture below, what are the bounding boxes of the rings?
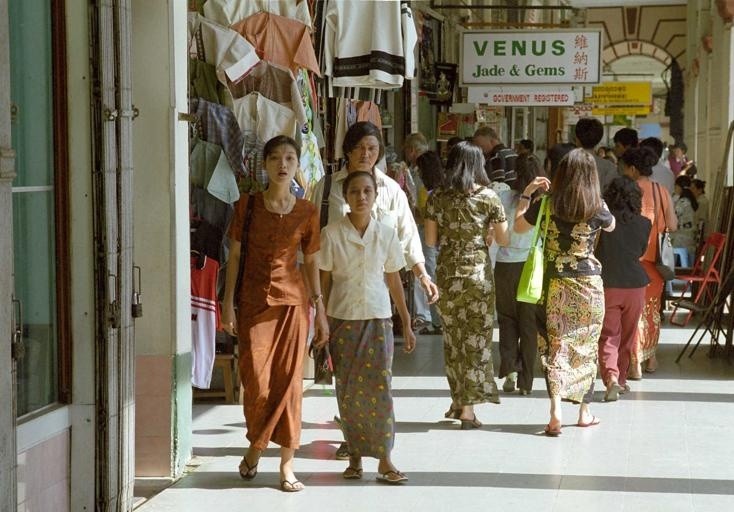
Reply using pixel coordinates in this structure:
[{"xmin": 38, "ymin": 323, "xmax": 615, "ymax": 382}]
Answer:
[{"xmin": 227, "ymin": 320, "xmax": 234, "ymax": 329}]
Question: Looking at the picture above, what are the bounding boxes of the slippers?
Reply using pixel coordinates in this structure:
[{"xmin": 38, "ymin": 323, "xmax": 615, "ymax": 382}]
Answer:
[
  {"xmin": 445, "ymin": 363, "xmax": 660, "ymax": 434},
  {"xmin": 343, "ymin": 467, "xmax": 363, "ymax": 479},
  {"xmin": 376, "ymin": 470, "xmax": 407, "ymax": 483},
  {"xmin": 239, "ymin": 455, "xmax": 258, "ymax": 481},
  {"xmin": 279, "ymin": 478, "xmax": 304, "ymax": 491}
]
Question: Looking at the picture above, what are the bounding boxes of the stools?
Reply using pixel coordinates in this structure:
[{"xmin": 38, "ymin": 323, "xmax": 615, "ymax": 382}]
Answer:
[
  {"xmin": 667, "ymin": 246, "xmax": 692, "ymax": 294},
  {"xmin": 192, "ymin": 351, "xmax": 237, "ymax": 409}
]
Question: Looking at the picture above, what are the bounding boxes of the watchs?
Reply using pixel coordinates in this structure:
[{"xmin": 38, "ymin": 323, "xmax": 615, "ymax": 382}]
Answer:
[{"xmin": 310, "ymin": 293, "xmax": 324, "ymax": 308}]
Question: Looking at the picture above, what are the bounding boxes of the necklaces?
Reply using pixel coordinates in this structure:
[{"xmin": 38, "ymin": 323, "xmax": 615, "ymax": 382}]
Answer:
[{"xmin": 264, "ymin": 190, "xmax": 292, "ymax": 218}]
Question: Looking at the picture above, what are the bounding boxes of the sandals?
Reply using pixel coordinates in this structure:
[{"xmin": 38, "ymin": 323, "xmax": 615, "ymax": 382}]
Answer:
[{"xmin": 420, "ymin": 323, "xmax": 442, "ymax": 335}]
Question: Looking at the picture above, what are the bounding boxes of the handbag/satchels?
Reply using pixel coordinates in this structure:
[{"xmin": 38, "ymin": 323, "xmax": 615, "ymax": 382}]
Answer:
[
  {"xmin": 655, "ymin": 227, "xmax": 675, "ymax": 281},
  {"xmin": 516, "ymin": 245, "xmax": 547, "ymax": 305},
  {"xmin": 217, "ymin": 253, "xmax": 245, "ymax": 310},
  {"xmin": 308, "ymin": 332, "xmax": 334, "ymax": 385}
]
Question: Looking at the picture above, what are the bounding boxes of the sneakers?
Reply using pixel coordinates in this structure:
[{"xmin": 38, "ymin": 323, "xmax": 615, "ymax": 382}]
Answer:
[{"xmin": 336, "ymin": 442, "xmax": 350, "ymax": 460}]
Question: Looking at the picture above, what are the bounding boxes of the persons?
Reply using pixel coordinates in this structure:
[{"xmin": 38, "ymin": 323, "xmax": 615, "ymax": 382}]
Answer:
[
  {"xmin": 309, "ymin": 121, "xmax": 440, "ymax": 459},
  {"xmin": 317, "ymin": 170, "xmax": 416, "ymax": 482},
  {"xmin": 422, "ymin": 139, "xmax": 511, "ymax": 429},
  {"xmin": 406, "ymin": 119, "xmax": 710, "ymax": 396},
  {"xmin": 514, "ymin": 149, "xmax": 618, "ymax": 435},
  {"xmin": 219, "ymin": 134, "xmax": 330, "ymax": 493}
]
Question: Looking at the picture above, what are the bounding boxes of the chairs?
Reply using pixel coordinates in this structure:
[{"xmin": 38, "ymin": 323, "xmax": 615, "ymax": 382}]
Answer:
[
  {"xmin": 666, "ymin": 231, "xmax": 727, "ymax": 326},
  {"xmin": 671, "ymin": 264, "xmax": 734, "ymax": 364}
]
